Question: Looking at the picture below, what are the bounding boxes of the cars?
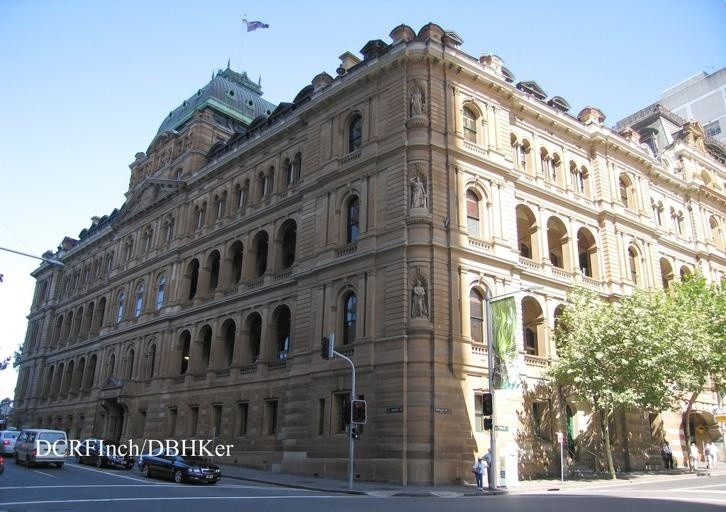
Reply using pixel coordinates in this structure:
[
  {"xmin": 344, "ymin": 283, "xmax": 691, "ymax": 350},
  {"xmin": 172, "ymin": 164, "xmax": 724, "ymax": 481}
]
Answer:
[
  {"xmin": 0, "ymin": 431, "xmax": 21, "ymax": 474},
  {"xmin": 74, "ymin": 438, "xmax": 221, "ymax": 483}
]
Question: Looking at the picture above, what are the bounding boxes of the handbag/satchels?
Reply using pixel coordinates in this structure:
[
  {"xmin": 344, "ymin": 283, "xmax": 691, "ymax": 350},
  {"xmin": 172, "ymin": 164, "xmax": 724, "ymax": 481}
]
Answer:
[{"xmin": 471, "ymin": 466, "xmax": 482, "ymax": 473}]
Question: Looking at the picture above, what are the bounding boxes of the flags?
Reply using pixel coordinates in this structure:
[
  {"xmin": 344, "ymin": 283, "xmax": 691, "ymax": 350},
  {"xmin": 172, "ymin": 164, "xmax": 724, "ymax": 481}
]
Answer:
[{"xmin": 243, "ymin": 19, "xmax": 271, "ymax": 33}]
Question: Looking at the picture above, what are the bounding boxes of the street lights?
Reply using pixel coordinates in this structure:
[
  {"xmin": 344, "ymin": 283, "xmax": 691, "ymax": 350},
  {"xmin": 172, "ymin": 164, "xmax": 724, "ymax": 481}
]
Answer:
[
  {"xmin": 0, "ymin": 246, "xmax": 66, "ymax": 268},
  {"xmin": 485, "ymin": 285, "xmax": 544, "ymax": 490}
]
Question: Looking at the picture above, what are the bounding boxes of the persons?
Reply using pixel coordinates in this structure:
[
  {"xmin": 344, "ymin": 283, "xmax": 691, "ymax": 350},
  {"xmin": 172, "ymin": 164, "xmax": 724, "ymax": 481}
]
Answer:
[
  {"xmin": 472, "ymin": 456, "xmax": 491, "ymax": 491},
  {"xmin": 411, "ymin": 87, "xmax": 424, "ymax": 116},
  {"xmin": 688, "ymin": 442, "xmax": 699, "ymax": 471},
  {"xmin": 662, "ymin": 441, "xmax": 673, "ymax": 470},
  {"xmin": 410, "ymin": 279, "xmax": 428, "ymax": 316},
  {"xmin": 702, "ymin": 442, "xmax": 711, "ymax": 469},
  {"xmin": 479, "ymin": 449, "xmax": 492, "ymax": 488},
  {"xmin": 409, "ymin": 175, "xmax": 426, "ymax": 207},
  {"xmin": 709, "ymin": 441, "xmax": 719, "ymax": 469}
]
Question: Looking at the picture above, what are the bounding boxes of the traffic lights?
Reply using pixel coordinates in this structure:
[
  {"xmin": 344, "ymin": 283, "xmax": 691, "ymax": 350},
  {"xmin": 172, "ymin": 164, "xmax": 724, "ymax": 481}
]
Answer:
[
  {"xmin": 353, "ymin": 402, "xmax": 365, "ymax": 421},
  {"xmin": 320, "ymin": 336, "xmax": 329, "ymax": 359},
  {"xmin": 352, "ymin": 428, "xmax": 358, "ymax": 439}
]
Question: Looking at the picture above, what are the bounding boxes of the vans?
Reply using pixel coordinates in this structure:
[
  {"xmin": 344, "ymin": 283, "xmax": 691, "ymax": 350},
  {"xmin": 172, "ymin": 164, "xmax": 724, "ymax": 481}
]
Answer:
[{"xmin": 12, "ymin": 428, "xmax": 68, "ymax": 468}]
